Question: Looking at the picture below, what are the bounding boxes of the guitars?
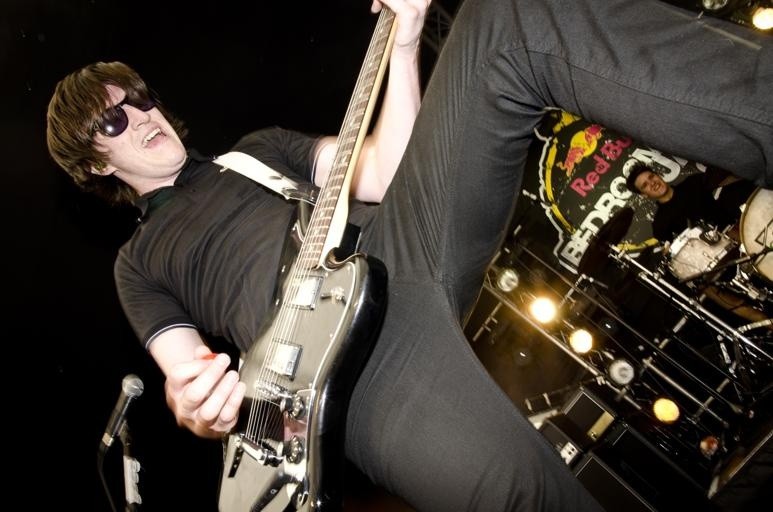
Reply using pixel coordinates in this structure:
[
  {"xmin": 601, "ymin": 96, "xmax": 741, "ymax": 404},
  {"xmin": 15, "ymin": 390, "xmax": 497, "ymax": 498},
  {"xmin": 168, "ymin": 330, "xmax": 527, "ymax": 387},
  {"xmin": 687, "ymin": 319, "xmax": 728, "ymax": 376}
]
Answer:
[{"xmin": 218, "ymin": 1, "xmax": 399, "ymax": 511}]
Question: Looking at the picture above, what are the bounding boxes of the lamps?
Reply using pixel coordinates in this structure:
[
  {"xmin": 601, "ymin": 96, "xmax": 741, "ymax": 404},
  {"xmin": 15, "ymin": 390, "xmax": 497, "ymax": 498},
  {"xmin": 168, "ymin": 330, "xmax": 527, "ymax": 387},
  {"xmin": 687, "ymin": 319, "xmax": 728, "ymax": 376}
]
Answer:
[{"xmin": 495, "ymin": 267, "xmax": 730, "ymax": 470}]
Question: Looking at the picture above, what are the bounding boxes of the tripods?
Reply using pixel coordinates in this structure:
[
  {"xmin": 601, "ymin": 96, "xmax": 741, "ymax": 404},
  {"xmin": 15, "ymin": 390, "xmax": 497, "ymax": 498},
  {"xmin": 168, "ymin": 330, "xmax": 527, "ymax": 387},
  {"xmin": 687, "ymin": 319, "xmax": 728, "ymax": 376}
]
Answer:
[{"xmin": 485, "ymin": 240, "xmax": 773, "ymax": 472}]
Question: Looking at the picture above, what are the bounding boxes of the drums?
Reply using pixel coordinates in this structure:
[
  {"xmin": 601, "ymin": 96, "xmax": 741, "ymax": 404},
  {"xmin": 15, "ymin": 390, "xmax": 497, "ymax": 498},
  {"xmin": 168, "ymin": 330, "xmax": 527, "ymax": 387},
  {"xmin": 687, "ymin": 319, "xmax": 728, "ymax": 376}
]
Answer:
[
  {"xmin": 663, "ymin": 218, "xmax": 737, "ymax": 291},
  {"xmin": 738, "ymin": 184, "xmax": 773, "ymax": 294}
]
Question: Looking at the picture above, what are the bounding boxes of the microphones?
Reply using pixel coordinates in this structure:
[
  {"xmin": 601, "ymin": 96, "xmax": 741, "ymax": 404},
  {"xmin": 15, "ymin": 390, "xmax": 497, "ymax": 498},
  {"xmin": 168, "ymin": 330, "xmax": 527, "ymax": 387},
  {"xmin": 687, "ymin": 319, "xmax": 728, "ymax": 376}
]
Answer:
[{"xmin": 101, "ymin": 374, "xmax": 144, "ymax": 447}]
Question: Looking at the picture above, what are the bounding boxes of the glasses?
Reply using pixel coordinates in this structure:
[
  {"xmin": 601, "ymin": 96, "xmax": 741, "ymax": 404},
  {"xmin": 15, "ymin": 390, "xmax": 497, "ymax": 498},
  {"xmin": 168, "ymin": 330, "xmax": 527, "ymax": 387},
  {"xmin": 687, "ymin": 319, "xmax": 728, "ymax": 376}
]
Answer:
[{"xmin": 87, "ymin": 88, "xmax": 155, "ymax": 145}]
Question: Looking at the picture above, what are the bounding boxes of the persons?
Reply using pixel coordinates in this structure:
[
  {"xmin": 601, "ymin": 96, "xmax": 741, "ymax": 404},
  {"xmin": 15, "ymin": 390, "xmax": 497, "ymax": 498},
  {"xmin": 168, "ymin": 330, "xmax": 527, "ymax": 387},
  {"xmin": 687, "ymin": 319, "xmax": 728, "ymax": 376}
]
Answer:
[
  {"xmin": 625, "ymin": 161, "xmax": 772, "ymax": 331},
  {"xmin": 47, "ymin": 1, "xmax": 773, "ymax": 511}
]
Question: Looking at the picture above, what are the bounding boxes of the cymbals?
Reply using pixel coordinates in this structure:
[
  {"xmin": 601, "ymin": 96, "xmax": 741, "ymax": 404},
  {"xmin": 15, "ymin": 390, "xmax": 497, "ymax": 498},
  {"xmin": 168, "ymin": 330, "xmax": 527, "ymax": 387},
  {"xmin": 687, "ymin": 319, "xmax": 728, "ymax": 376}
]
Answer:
[{"xmin": 577, "ymin": 206, "xmax": 633, "ymax": 274}]
